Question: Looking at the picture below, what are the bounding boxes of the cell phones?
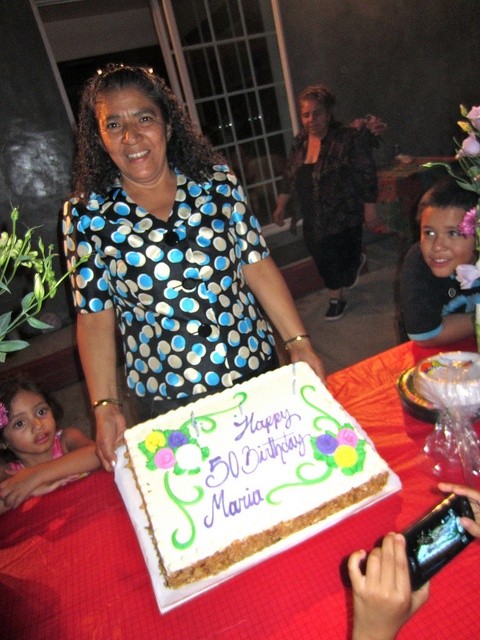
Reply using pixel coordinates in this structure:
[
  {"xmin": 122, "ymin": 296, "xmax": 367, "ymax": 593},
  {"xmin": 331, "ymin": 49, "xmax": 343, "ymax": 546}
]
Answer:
[{"xmin": 402, "ymin": 494, "xmax": 476, "ymax": 592}]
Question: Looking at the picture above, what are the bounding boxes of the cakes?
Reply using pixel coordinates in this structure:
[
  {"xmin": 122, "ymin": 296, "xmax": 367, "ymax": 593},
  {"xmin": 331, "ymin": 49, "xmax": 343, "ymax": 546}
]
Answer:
[
  {"xmin": 416, "ymin": 507, "xmax": 466, "ymax": 567},
  {"xmin": 122, "ymin": 361, "xmax": 390, "ymax": 590}
]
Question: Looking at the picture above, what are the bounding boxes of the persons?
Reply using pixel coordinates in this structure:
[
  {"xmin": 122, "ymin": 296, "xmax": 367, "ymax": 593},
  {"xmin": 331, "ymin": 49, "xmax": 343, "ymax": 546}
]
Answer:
[
  {"xmin": 399, "ymin": 178, "xmax": 480, "ymax": 348},
  {"xmin": 347, "ymin": 481, "xmax": 480, "ymax": 640},
  {"xmin": 271, "ymin": 84, "xmax": 382, "ymax": 321},
  {"xmin": 0, "ymin": 371, "xmax": 102, "ymax": 515},
  {"xmin": 60, "ymin": 61, "xmax": 327, "ymax": 474}
]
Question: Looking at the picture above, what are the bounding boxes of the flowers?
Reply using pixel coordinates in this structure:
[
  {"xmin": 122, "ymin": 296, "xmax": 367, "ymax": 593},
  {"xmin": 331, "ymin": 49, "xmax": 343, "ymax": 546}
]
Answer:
[
  {"xmin": 345, "ymin": 113, "xmax": 397, "ymax": 160},
  {"xmin": 0, "ymin": 208, "xmax": 89, "ymax": 364},
  {"xmin": 421, "ymin": 104, "xmax": 480, "ymax": 289}
]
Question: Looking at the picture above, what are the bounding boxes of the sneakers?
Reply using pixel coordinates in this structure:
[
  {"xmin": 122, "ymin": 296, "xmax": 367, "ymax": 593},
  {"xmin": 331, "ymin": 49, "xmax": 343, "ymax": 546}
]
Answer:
[
  {"xmin": 324, "ymin": 296, "xmax": 348, "ymax": 321},
  {"xmin": 345, "ymin": 252, "xmax": 366, "ymax": 290}
]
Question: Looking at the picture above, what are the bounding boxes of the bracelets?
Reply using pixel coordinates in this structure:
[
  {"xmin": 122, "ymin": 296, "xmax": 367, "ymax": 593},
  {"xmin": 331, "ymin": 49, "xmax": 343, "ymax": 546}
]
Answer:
[
  {"xmin": 89, "ymin": 398, "xmax": 123, "ymax": 414},
  {"xmin": 280, "ymin": 333, "xmax": 311, "ymax": 351}
]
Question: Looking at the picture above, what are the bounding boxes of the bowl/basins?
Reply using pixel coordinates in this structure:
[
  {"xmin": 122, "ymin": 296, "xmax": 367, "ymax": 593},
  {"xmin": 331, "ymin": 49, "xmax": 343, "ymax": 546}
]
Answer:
[
  {"xmin": 396, "ymin": 368, "xmax": 479, "ymax": 426},
  {"xmin": 418, "ymin": 350, "xmax": 479, "ymax": 403}
]
